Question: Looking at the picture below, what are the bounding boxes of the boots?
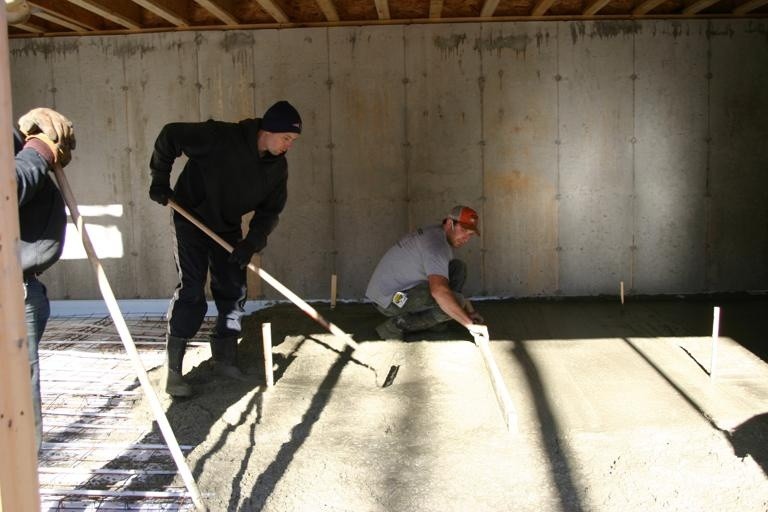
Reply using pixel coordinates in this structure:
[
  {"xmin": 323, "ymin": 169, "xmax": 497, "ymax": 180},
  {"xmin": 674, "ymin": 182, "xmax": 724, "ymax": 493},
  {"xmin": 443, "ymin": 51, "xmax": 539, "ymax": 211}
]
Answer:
[
  {"xmin": 160, "ymin": 333, "xmax": 192, "ymax": 397},
  {"xmin": 209, "ymin": 332, "xmax": 256, "ymax": 383}
]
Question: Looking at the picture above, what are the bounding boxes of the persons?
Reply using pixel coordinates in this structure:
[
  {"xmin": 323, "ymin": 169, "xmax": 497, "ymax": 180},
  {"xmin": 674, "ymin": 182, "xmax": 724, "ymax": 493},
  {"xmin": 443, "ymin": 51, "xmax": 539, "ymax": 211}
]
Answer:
[
  {"xmin": 11, "ymin": 108, "xmax": 77, "ymax": 454},
  {"xmin": 365, "ymin": 206, "xmax": 491, "ymax": 346},
  {"xmin": 149, "ymin": 101, "xmax": 302, "ymax": 398}
]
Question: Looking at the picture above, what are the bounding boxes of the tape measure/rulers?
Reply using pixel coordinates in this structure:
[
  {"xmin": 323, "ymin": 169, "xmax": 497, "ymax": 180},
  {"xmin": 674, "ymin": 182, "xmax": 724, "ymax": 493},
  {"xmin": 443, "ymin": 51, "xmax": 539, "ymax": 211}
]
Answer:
[{"xmin": 392, "ymin": 292, "xmax": 407, "ymax": 309}]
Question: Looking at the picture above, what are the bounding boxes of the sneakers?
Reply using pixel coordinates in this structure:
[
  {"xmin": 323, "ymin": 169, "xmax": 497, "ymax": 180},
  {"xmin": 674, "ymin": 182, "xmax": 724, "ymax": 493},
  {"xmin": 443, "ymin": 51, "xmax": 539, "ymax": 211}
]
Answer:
[{"xmin": 374, "ymin": 315, "xmax": 405, "ymax": 342}]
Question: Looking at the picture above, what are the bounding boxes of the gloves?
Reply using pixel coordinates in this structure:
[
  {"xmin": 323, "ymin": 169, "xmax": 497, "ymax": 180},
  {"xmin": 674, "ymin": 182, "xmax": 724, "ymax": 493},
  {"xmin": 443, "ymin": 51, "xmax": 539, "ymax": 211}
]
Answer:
[
  {"xmin": 147, "ymin": 176, "xmax": 176, "ymax": 206},
  {"xmin": 226, "ymin": 238, "xmax": 254, "ymax": 270},
  {"xmin": 17, "ymin": 105, "xmax": 77, "ymax": 168}
]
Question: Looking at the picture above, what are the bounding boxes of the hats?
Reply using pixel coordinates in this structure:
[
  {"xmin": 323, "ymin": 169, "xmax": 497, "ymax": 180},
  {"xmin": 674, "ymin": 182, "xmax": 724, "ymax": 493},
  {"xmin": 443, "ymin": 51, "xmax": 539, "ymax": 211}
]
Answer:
[
  {"xmin": 260, "ymin": 100, "xmax": 302, "ymax": 136},
  {"xmin": 447, "ymin": 204, "xmax": 484, "ymax": 236}
]
